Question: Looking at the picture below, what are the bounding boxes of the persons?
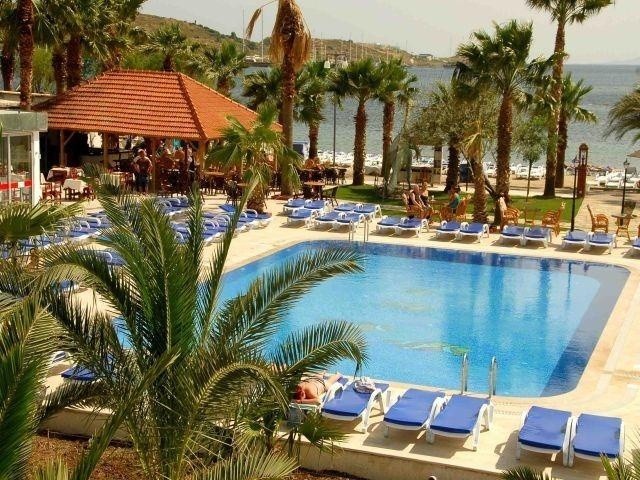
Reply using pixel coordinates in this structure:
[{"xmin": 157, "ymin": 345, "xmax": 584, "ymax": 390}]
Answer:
[
  {"xmin": 419, "ymin": 182, "xmax": 433, "ymax": 219},
  {"xmin": 408, "ymin": 184, "xmax": 426, "ymax": 219},
  {"xmin": 441, "ymin": 184, "xmax": 460, "ymax": 222},
  {"xmin": 291, "ymin": 369, "xmax": 342, "ymax": 403},
  {"xmin": 131, "ymin": 145, "xmax": 325, "ymax": 201}
]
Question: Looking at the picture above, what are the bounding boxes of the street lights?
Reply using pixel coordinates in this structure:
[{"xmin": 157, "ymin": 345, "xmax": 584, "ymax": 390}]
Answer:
[
  {"xmin": 324, "ymin": 53, "xmax": 348, "ymax": 165},
  {"xmin": 620, "ymin": 158, "xmax": 630, "ymax": 226},
  {"xmin": 570, "ymin": 155, "xmax": 580, "ymax": 232}
]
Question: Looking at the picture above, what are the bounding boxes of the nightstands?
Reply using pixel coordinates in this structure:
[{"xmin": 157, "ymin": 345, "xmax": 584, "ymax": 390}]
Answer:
[{"xmin": 632, "ymin": 235, "xmax": 640, "ymax": 253}]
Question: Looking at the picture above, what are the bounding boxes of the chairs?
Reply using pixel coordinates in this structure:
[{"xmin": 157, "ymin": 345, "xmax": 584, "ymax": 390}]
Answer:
[
  {"xmin": 1, "ymin": 196, "xmax": 272, "ymax": 293},
  {"xmin": 283, "ymin": 189, "xmax": 384, "ymax": 256},
  {"xmin": 376, "ymin": 214, "xmax": 490, "ymax": 244},
  {"xmin": 289, "ymin": 374, "xmax": 394, "ymax": 434},
  {"xmin": 562, "ymin": 229, "xmax": 619, "ymax": 255},
  {"xmin": 378, "ymin": 386, "xmax": 495, "ymax": 452},
  {"xmin": 499, "ymin": 224, "xmax": 553, "ymax": 250},
  {"xmin": 585, "ymin": 203, "xmax": 609, "ymax": 232},
  {"xmin": 401, "ymin": 189, "xmax": 563, "ymax": 237},
  {"xmin": 1, "ymin": 166, "xmax": 347, "ymax": 208},
  {"xmin": 62, "ymin": 351, "xmax": 112, "ymax": 384},
  {"xmin": 515, "ymin": 405, "xmax": 627, "ymax": 472},
  {"xmin": 585, "ymin": 171, "xmax": 640, "ymax": 189}
]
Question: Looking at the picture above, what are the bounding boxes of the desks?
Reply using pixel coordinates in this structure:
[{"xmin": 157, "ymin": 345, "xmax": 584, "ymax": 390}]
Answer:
[{"xmin": 612, "ymin": 212, "xmax": 638, "ymax": 242}]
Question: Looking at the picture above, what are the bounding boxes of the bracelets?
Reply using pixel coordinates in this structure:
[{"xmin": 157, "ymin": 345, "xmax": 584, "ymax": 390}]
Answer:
[{"xmin": 301, "ymin": 401, "xmax": 302, "ymax": 403}]
